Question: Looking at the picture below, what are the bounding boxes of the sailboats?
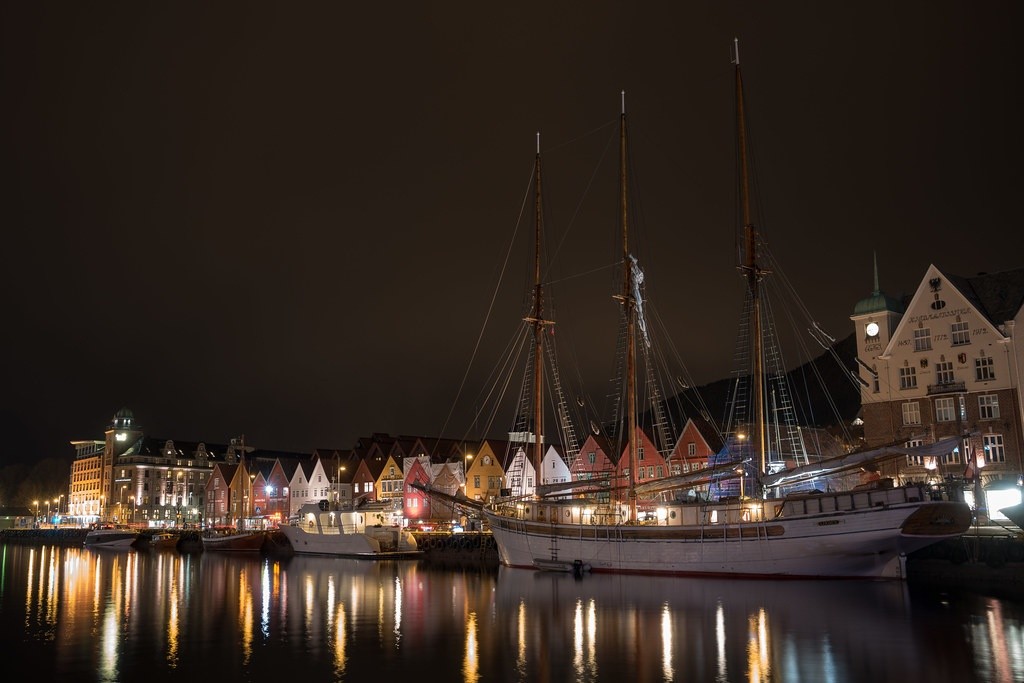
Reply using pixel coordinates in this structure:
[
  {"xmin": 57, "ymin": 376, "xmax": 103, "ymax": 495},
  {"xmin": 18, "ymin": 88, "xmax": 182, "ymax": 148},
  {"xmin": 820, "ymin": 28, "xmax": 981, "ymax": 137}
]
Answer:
[{"xmin": 408, "ymin": 38, "xmax": 988, "ymax": 582}]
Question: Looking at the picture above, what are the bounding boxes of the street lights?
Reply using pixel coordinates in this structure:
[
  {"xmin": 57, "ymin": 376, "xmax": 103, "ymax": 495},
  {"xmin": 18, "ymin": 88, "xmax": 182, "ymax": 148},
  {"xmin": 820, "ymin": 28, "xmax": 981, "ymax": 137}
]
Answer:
[
  {"xmin": 44, "ymin": 500, "xmax": 50, "ymax": 524},
  {"xmin": 116, "ymin": 486, "xmax": 127, "ymax": 520},
  {"xmin": 737, "ymin": 433, "xmax": 745, "ymax": 500},
  {"xmin": 32, "ymin": 501, "xmax": 39, "ymax": 516},
  {"xmin": 464, "ymin": 454, "xmax": 472, "ymax": 497},
  {"xmin": 337, "ymin": 467, "xmax": 345, "ymax": 503},
  {"xmin": 57, "ymin": 494, "xmax": 64, "ymax": 513},
  {"xmin": 130, "ymin": 496, "xmax": 136, "ymax": 521}
]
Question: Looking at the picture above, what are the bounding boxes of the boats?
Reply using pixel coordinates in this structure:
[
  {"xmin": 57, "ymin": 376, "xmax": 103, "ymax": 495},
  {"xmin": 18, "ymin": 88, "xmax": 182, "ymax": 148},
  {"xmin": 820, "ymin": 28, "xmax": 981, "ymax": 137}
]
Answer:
[
  {"xmin": 149, "ymin": 528, "xmax": 181, "ymax": 548},
  {"xmin": 531, "ymin": 558, "xmax": 572, "ymax": 572},
  {"xmin": 201, "ymin": 529, "xmax": 268, "ymax": 551},
  {"xmin": 276, "ymin": 496, "xmax": 427, "ymax": 557},
  {"xmin": 82, "ymin": 521, "xmax": 142, "ymax": 547}
]
